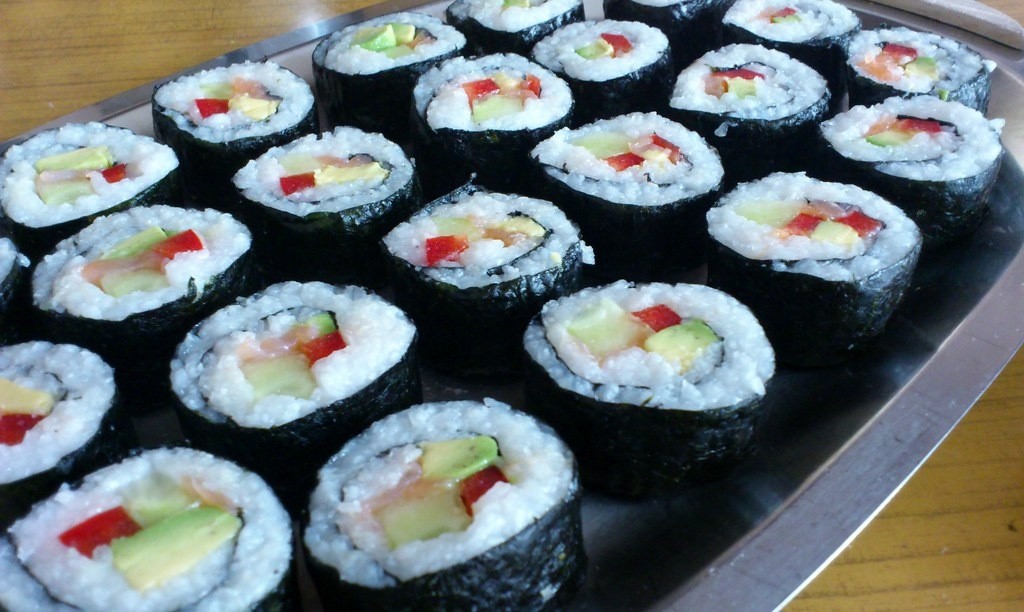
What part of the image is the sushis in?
[0,0,1004,612]
